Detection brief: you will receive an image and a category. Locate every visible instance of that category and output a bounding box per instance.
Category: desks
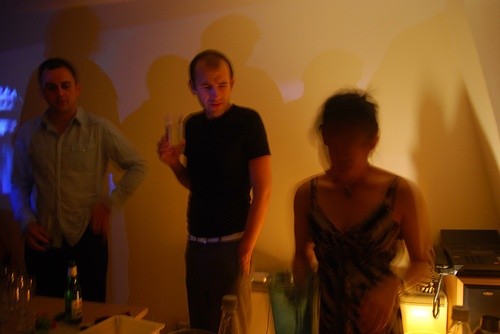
[0,297,149,334]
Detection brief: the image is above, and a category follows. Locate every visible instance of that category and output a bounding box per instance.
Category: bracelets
[397,278,405,295]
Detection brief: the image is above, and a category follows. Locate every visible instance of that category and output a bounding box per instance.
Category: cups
[165,113,183,153]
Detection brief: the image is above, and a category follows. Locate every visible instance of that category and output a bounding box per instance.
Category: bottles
[446,305,473,334]
[64,258,83,324]
[218,295,240,334]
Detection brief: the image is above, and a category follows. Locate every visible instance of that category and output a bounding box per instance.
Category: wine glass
[0,266,35,320]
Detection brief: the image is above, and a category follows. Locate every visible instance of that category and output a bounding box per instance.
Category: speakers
[456,283,500,334]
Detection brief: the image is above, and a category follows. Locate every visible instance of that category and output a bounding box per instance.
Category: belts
[189,231,245,243]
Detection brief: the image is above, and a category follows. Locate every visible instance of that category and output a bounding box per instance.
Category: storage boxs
[78,314,165,334]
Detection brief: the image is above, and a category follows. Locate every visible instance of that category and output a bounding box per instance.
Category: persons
[155,49,273,334]
[11,57,150,306]
[289,88,430,334]
